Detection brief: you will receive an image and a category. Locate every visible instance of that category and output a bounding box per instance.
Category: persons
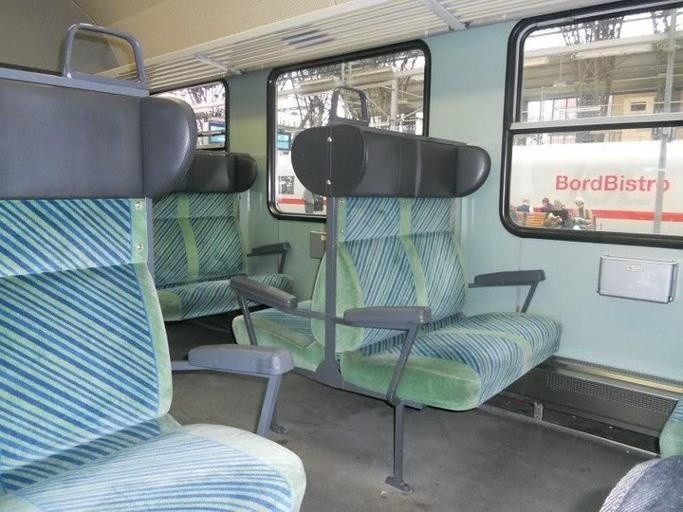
[572,199,593,230]
[301,188,314,214]
[539,197,555,212]
[540,199,571,229]
[514,197,534,212]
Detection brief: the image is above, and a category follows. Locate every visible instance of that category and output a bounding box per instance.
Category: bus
[196,112,309,219]
